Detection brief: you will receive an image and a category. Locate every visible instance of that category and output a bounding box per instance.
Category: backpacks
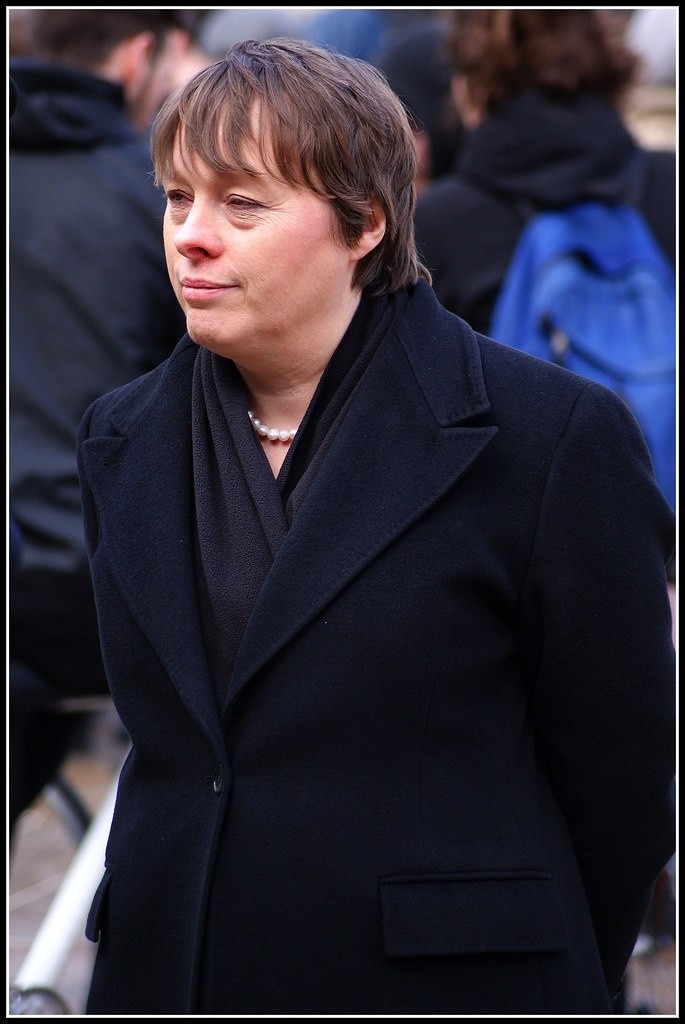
[486,147,676,514]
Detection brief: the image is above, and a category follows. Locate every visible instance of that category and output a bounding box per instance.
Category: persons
[379,28,478,198]
[8,6,192,868]
[413,10,681,965]
[74,36,677,1017]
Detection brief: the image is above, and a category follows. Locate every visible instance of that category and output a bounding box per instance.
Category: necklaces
[246,409,297,441]
